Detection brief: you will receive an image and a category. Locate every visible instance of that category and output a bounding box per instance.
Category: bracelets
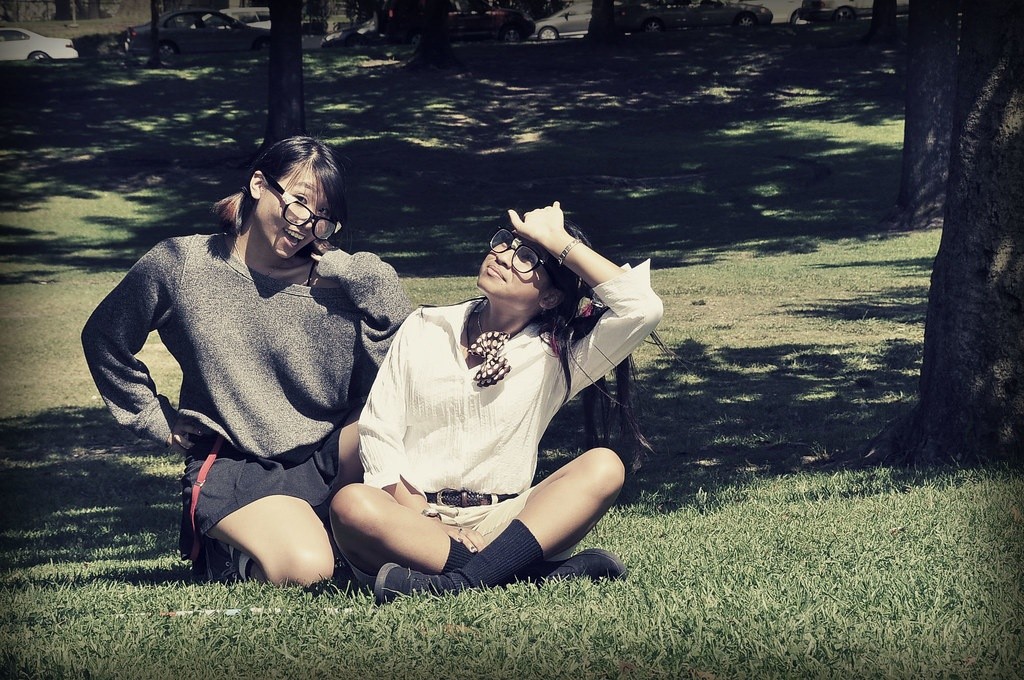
[558,239,583,266]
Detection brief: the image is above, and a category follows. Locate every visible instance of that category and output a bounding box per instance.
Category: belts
[424,491,518,507]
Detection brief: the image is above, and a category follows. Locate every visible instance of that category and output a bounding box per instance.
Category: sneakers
[374,563,443,604]
[205,537,244,585]
[535,549,627,590]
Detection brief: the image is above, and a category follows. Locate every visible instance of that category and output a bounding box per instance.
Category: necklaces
[477,313,484,334]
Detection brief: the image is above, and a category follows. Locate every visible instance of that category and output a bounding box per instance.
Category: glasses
[262,169,337,241]
[490,225,556,282]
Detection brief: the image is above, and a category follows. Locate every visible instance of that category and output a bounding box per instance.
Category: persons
[80,135,417,589]
[331,201,665,605]
